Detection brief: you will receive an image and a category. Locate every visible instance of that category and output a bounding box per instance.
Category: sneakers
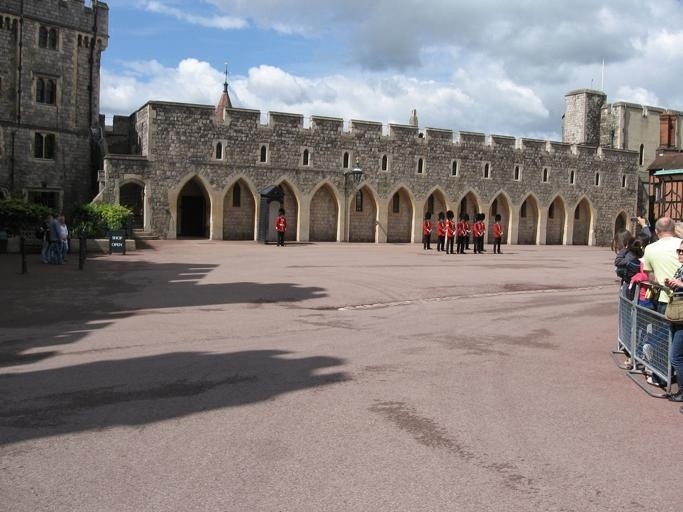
[615,356,683,403]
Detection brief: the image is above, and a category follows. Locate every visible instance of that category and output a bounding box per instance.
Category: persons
[608,212,682,415]
[37,213,69,265]
[272,213,288,247]
[421,218,504,254]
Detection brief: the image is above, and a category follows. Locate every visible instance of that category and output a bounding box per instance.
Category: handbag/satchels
[663,298,683,325]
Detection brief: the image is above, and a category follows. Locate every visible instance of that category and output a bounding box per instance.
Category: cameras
[630,217,639,225]
